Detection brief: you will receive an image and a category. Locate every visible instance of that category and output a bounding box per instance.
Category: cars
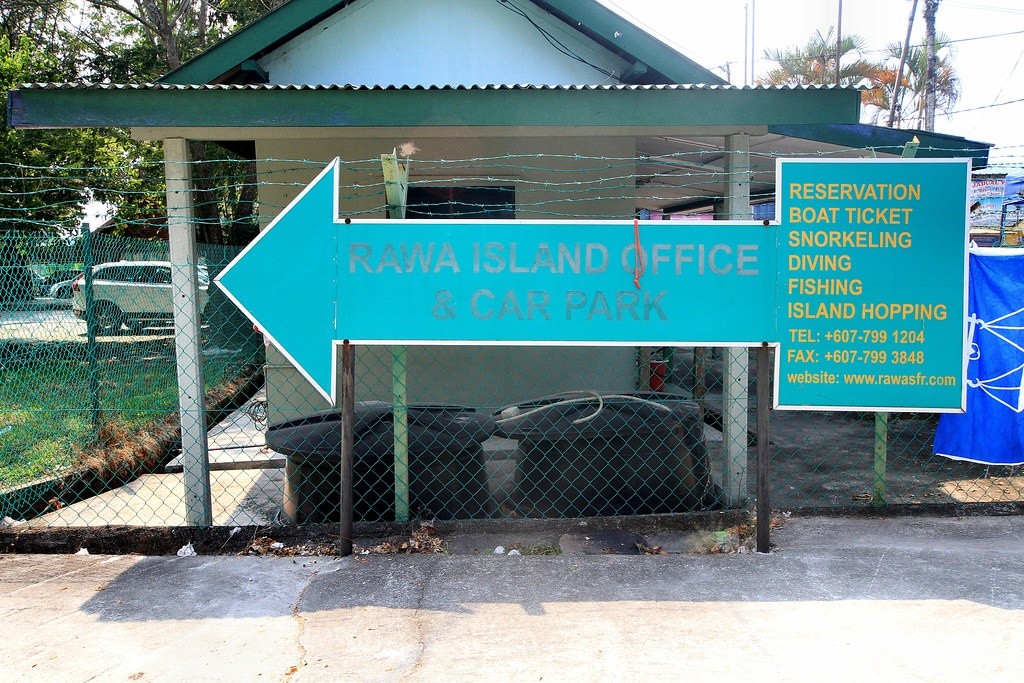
[25,269,86,299]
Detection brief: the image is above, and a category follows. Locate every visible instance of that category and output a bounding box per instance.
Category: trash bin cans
[262,402,497,526]
[490,394,723,515]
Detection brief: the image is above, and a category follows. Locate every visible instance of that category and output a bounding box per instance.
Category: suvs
[71,260,213,335]
